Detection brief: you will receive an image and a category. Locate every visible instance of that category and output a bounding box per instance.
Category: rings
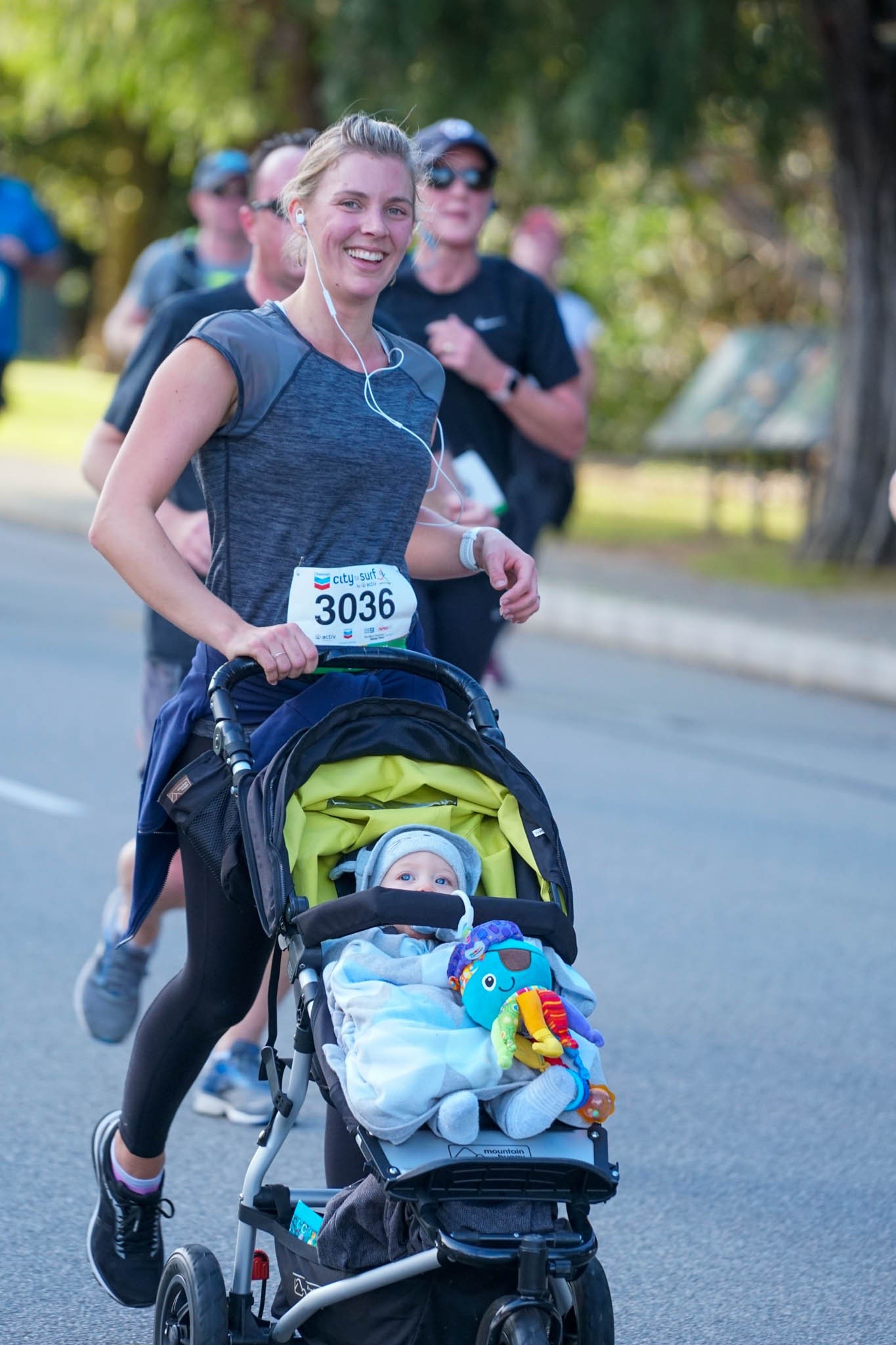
[274,651,287,659]
[537,593,541,601]
[442,341,454,354]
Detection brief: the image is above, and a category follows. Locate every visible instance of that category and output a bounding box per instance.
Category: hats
[412,117,499,173]
[188,148,249,190]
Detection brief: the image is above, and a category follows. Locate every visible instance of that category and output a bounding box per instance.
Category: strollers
[153,645,621,1344]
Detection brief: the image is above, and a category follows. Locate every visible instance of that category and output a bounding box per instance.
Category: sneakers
[192,1040,277,1124]
[77,889,155,1046]
[87,1107,174,1309]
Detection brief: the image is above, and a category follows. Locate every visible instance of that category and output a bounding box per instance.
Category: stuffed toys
[445,918,603,1079]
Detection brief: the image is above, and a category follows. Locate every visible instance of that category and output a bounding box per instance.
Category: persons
[89,114,543,1307]
[94,115,591,709]
[334,825,573,1143]
[46,114,507,1127]
[0,174,65,416]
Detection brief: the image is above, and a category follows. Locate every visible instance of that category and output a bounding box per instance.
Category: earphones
[296,214,305,224]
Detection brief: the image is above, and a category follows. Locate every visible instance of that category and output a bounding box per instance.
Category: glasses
[216,186,247,198]
[249,197,283,216]
[426,165,491,190]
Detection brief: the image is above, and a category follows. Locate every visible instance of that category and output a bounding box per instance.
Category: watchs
[488,367,520,403]
[458,526,487,572]
